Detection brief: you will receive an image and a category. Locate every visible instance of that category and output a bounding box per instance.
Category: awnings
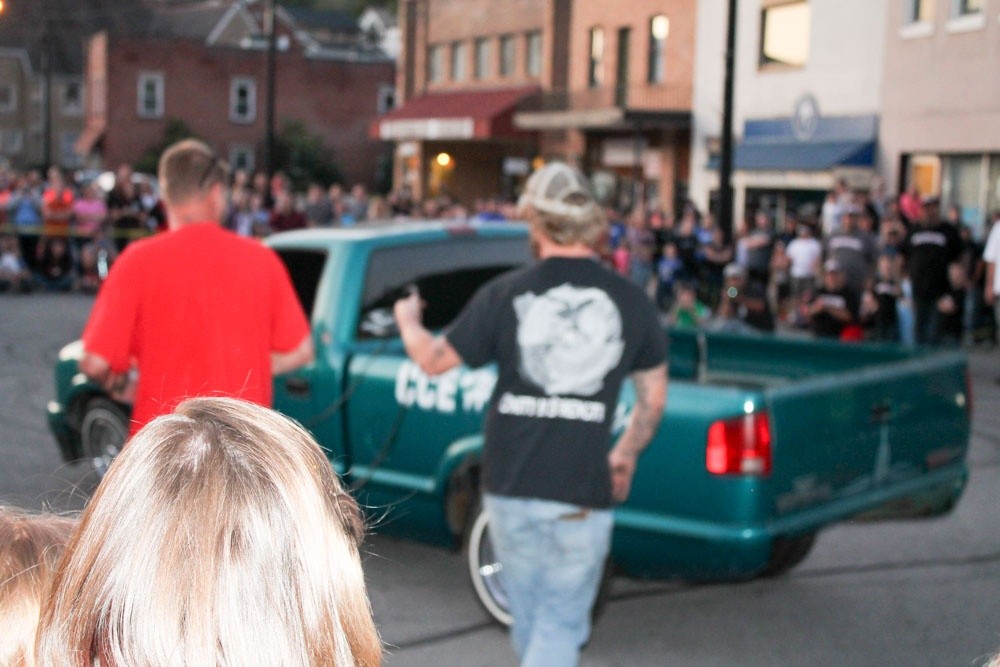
[704,115,877,171]
[371,86,543,141]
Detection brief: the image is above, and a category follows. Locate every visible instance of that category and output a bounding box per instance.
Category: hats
[515,161,595,217]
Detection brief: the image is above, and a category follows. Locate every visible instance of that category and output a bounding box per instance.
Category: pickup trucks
[41,218,977,637]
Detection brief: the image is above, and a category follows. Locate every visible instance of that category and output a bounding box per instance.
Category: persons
[393,160,668,667]
[81,138,314,441]
[606,178,999,389]
[0,396,383,667]
[0,157,516,296]
